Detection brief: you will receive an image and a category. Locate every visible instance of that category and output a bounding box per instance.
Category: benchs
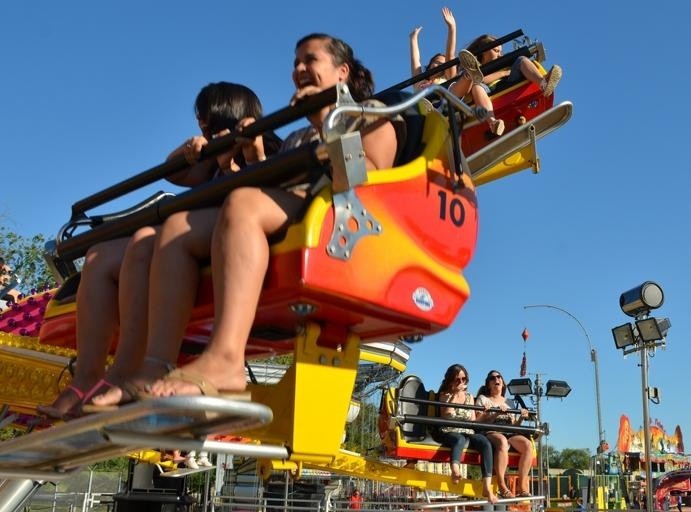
[39,91,479,368]
[379,375,541,469]
[376,29,572,190]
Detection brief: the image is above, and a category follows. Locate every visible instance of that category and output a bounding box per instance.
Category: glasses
[488,375,501,381]
[454,377,467,383]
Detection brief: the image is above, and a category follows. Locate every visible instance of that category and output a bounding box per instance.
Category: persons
[349,490,363,509]
[466,34,562,136]
[80,32,400,416]
[576,498,584,511]
[677,496,682,512]
[160,448,190,465]
[1,280,62,307]
[475,370,535,498]
[184,450,213,470]
[37,80,284,423]
[437,364,498,505]
[410,7,483,110]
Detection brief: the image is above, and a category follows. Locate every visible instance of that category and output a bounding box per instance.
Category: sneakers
[172,456,191,464]
[490,118,507,137]
[184,457,200,470]
[458,49,485,85]
[538,64,563,98]
[160,454,173,462]
[197,456,213,468]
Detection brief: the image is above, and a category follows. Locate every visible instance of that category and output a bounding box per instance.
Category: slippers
[516,490,531,498]
[39,379,87,419]
[137,367,254,408]
[68,377,119,417]
[499,488,515,499]
[79,380,145,414]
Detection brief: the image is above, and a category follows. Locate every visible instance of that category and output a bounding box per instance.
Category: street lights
[524,304,606,458]
[506,371,571,505]
[612,280,673,512]
